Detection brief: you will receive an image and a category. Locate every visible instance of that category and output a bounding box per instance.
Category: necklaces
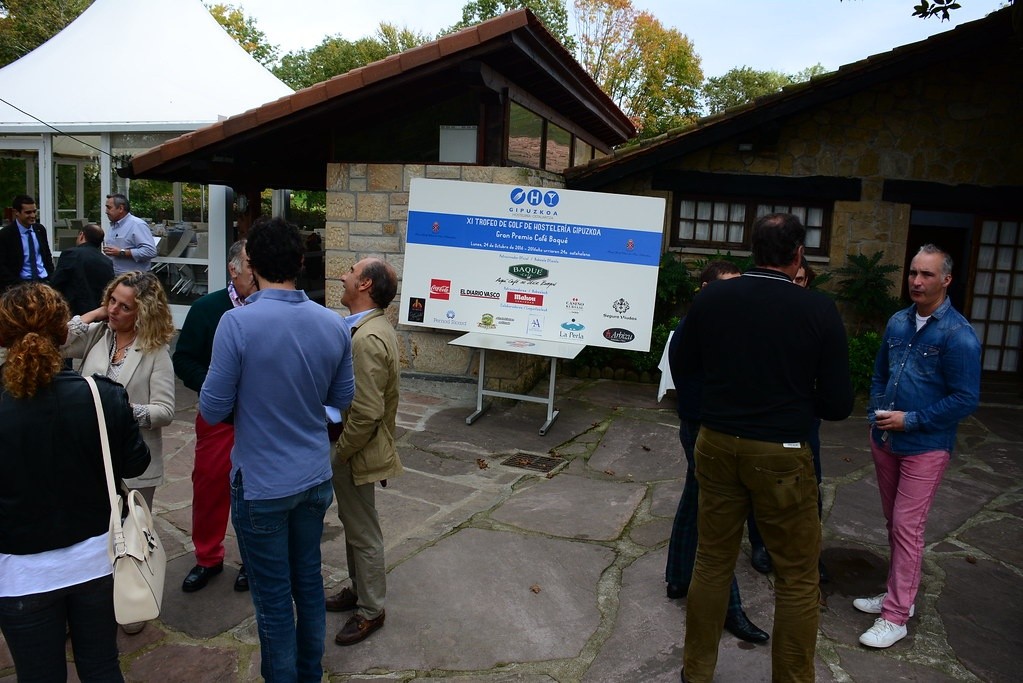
[114,336,136,360]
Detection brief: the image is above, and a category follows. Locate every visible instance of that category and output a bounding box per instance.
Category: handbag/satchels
[107,487,166,625]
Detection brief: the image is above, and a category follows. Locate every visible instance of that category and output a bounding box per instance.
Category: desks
[448,333,586,435]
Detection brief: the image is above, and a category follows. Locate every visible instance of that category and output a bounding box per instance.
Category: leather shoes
[182,560,224,592]
[722,610,770,643]
[234,565,250,591]
[666,577,691,599]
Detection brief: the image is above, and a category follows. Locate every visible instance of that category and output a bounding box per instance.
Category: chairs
[55,218,208,296]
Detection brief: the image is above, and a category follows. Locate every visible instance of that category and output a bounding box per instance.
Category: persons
[200,217,356,683]
[852,244,981,648]
[60,270,176,634]
[173,240,251,593]
[668,212,853,683]
[103,195,157,277]
[665,262,770,643]
[49,224,114,370]
[0,195,55,298]
[326,259,403,646]
[0,283,151,683]
[237,215,248,240]
[298,233,324,292]
[747,256,835,584]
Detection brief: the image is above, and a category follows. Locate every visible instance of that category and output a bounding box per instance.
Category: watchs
[121,248,125,257]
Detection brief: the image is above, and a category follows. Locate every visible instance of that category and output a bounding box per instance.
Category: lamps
[737,139,753,151]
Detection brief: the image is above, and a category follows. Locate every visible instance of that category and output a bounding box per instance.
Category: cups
[103,242,110,251]
[874,394,891,418]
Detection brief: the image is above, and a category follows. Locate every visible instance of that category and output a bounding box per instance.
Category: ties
[25,230,39,283]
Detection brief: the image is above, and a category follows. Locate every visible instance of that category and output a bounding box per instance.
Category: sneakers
[859,618,908,650]
[750,538,775,573]
[853,592,916,618]
[335,609,386,646]
[324,587,358,611]
[818,561,828,578]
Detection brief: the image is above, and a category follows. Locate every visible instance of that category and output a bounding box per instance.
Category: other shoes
[121,622,145,634]
